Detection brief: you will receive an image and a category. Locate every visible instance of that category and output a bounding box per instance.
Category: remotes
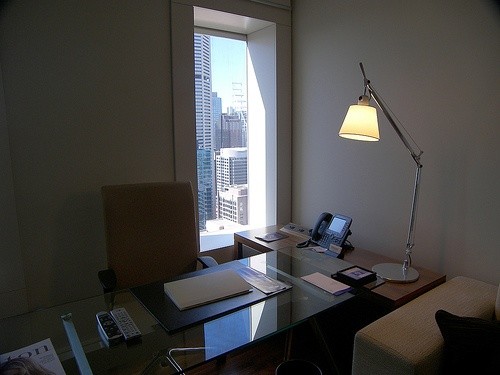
[96,311,122,340]
[111,308,142,339]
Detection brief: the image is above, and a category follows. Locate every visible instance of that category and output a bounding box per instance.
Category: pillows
[434,309,500,375]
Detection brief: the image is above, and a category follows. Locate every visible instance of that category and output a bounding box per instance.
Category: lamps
[338,61,424,283]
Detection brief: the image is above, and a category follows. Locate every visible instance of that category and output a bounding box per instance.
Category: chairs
[99,179,220,297]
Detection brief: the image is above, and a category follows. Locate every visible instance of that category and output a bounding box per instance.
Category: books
[0,338,66,375]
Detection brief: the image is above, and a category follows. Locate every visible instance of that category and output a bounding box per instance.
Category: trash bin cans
[276,359,323,375]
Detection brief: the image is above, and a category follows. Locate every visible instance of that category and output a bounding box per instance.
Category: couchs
[351,276,500,375]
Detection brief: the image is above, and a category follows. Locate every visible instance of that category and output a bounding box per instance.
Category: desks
[0,246,385,375]
[234,224,447,307]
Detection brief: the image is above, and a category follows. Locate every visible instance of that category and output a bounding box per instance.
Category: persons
[0,356,56,375]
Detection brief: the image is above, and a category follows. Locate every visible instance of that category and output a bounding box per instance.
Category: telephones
[310,212,352,249]
